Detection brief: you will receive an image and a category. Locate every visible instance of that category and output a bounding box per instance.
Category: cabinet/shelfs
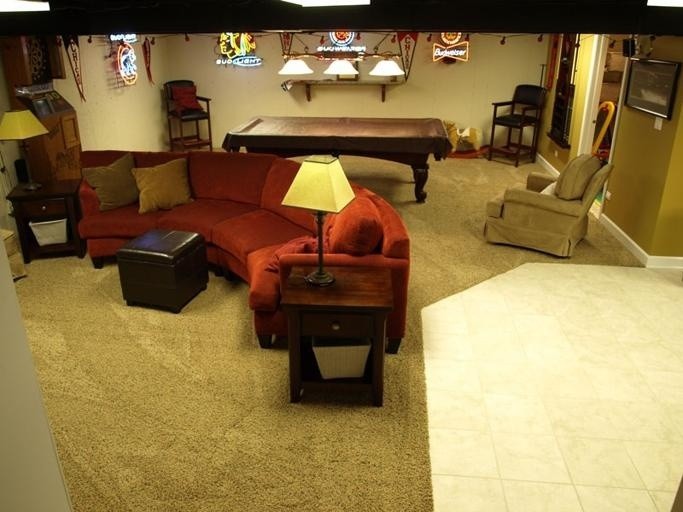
[6,177,87,264]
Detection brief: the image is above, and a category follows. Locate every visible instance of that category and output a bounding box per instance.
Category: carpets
[13,154,646,512]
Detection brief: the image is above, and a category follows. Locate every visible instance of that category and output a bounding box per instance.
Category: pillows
[131,157,194,215]
[324,190,383,254]
[266,234,317,272]
[81,152,140,211]
[540,180,557,196]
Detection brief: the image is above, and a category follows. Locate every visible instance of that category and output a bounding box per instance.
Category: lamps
[369,60,405,76]
[280,155,356,286]
[323,60,359,75]
[0,110,50,191]
[278,58,314,75]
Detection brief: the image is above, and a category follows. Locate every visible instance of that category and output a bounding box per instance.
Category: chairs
[163,79,213,152]
[489,84,547,167]
[482,153,614,257]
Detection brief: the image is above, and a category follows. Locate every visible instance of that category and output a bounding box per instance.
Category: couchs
[78,150,410,354]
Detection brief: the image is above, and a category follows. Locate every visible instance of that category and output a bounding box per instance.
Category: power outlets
[653,117,663,131]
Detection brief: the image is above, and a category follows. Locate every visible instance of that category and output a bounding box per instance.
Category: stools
[116,228,209,313]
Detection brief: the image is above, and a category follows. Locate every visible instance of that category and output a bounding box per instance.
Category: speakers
[623,39,635,57]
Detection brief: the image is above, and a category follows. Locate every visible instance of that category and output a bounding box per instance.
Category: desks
[222,115,454,203]
[280,265,394,407]
